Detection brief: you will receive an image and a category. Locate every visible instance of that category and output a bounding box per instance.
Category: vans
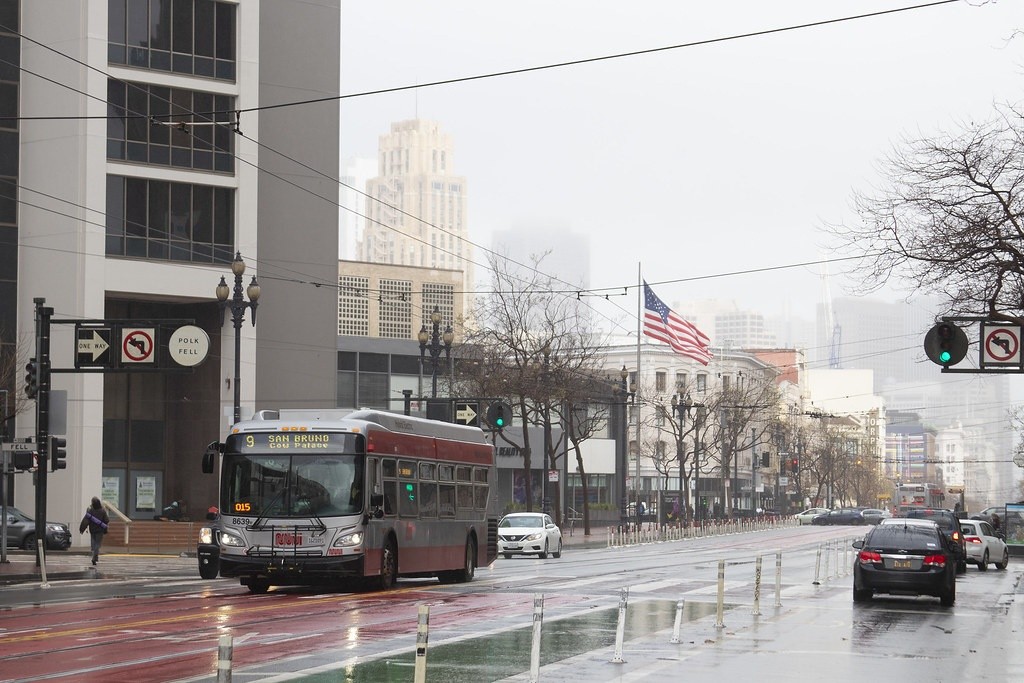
[906,506,970,574]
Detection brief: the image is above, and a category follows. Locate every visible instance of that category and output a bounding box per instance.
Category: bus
[202,407,499,593]
[896,484,945,520]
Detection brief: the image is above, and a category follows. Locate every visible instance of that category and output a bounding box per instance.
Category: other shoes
[91,559,98,565]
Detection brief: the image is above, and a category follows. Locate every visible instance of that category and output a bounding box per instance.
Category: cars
[793,507,893,526]
[497,513,563,559]
[852,523,958,606]
[957,520,1009,571]
[0,505,73,551]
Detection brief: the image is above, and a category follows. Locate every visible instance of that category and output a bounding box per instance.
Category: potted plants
[582,502,621,527]
[505,501,541,514]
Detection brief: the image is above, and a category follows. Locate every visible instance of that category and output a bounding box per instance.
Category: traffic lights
[792,458,798,473]
[486,402,513,428]
[924,321,968,367]
[25,358,36,399]
[51,437,66,471]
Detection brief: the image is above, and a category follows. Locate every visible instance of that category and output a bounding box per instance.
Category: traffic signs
[77,328,111,366]
[456,403,480,427]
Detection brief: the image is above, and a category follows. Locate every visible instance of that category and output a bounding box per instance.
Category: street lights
[418,303,454,397]
[671,382,692,521]
[727,411,745,515]
[613,365,639,533]
[216,249,261,426]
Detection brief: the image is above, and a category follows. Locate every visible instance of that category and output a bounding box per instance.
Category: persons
[334,473,362,513]
[759,505,766,516]
[79,496,109,565]
[989,514,1000,529]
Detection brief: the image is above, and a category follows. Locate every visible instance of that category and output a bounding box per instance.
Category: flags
[643,279,714,366]
